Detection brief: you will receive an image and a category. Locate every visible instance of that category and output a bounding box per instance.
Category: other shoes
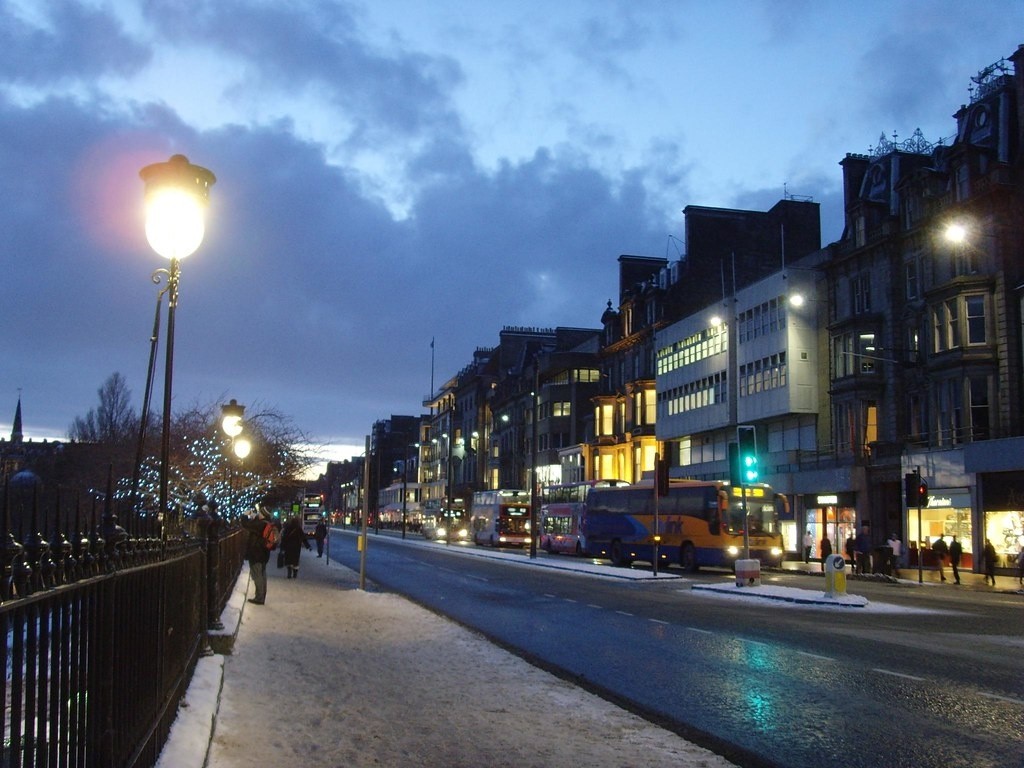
[248,599,264,605]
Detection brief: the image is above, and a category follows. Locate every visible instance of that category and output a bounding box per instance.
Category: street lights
[140,153,218,558]
[221,398,250,536]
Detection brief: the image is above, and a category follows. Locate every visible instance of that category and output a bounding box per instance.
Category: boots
[293,568,299,577]
[287,565,292,578]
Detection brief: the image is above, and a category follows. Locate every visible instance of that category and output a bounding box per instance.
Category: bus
[580,478,790,572]
[471,488,531,547]
[299,492,327,538]
[421,504,468,540]
[540,479,631,557]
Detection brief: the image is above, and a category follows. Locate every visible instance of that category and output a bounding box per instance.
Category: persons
[803,525,1024,586]
[271,516,312,578]
[314,519,327,558]
[241,505,272,605]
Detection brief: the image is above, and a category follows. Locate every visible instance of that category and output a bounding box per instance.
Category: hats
[259,506,272,516]
[208,500,219,510]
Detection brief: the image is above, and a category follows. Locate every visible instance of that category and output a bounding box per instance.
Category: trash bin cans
[855,552,870,573]
[873,546,894,574]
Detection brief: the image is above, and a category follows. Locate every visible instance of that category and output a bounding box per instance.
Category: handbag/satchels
[278,553,286,569]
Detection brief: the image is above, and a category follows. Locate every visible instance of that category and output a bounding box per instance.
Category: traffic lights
[736,425,758,485]
[919,485,928,506]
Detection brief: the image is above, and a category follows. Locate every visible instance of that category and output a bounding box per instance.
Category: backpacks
[262,520,279,550]
